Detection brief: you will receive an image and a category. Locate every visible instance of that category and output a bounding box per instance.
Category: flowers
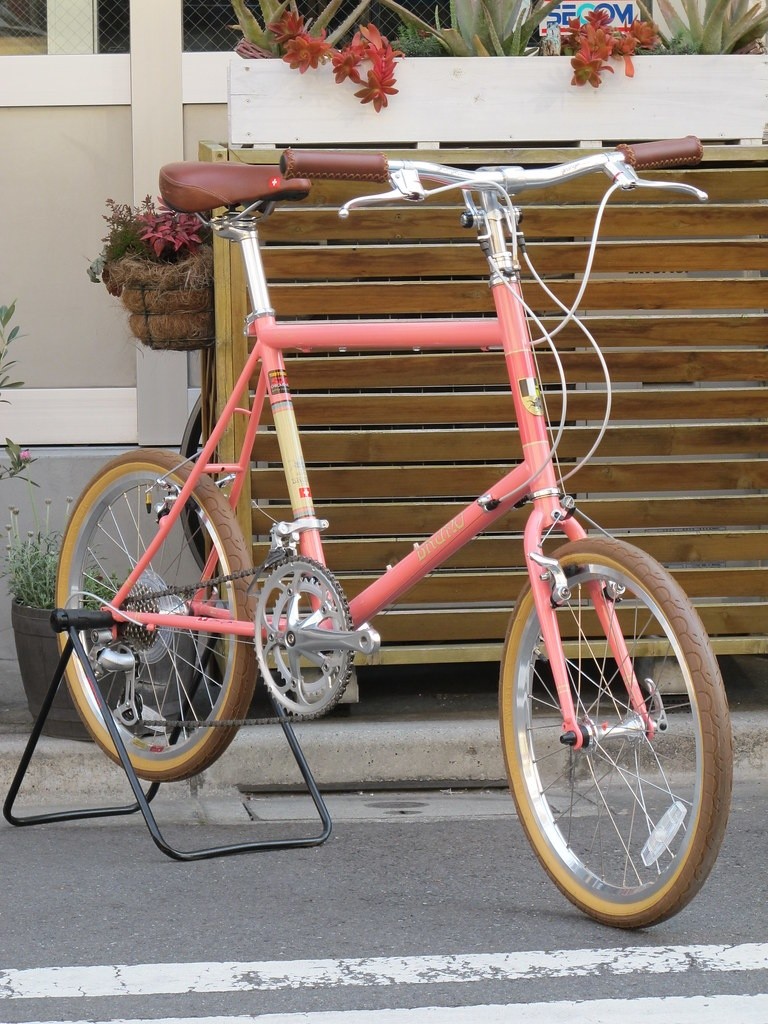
[2,450,140,612]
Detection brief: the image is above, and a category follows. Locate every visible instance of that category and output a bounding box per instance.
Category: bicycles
[3,133,733,930]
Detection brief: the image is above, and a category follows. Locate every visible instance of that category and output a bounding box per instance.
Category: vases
[10,591,126,744]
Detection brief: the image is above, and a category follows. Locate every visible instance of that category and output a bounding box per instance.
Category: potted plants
[226,0,768,145]
[100,192,218,353]
